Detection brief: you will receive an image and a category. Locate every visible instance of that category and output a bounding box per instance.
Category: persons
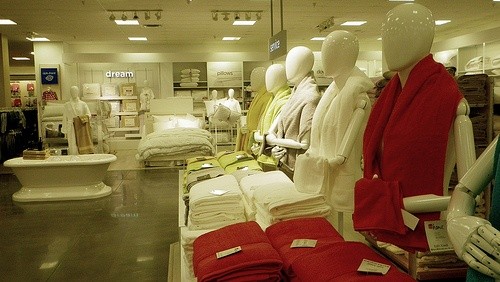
[266,46,321,172]
[42,87,59,105]
[363,3,476,234]
[211,90,218,101]
[224,88,241,114]
[251,63,291,156]
[297,30,371,204]
[140,80,154,103]
[240,66,271,148]
[445,133,500,282]
[61,86,91,155]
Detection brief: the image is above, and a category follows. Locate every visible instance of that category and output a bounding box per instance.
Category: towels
[23,149,50,160]
[247,83,292,156]
[258,70,321,178]
[73,115,94,154]
[293,65,376,212]
[182,151,417,282]
[234,87,272,154]
[352,54,464,253]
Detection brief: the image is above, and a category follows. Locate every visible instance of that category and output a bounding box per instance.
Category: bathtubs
[4,154,117,202]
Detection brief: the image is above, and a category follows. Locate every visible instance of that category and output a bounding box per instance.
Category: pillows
[186,113,196,118]
[181,74,200,77]
[180,69,200,74]
[177,118,200,129]
[181,77,199,82]
[153,119,175,131]
[211,103,242,126]
[152,114,175,122]
[170,115,202,125]
[179,82,198,87]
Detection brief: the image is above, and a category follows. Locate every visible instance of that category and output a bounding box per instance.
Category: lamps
[107,9,164,21]
[316,17,334,33]
[211,10,263,21]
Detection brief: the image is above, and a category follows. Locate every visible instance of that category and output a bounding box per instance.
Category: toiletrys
[27,137,47,151]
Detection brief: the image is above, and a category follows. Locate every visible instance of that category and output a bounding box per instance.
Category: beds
[135,113,217,167]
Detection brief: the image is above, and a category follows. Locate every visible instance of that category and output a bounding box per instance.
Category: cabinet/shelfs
[0,27,500,148]
[358,67,494,280]
